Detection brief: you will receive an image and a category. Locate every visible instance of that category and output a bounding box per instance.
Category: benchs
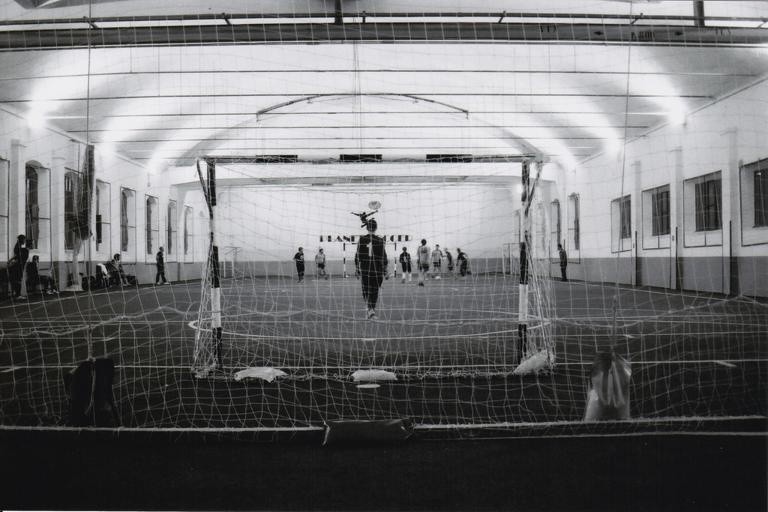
[96,263,136,288]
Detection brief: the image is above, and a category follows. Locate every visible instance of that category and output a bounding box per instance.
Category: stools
[26,279,36,292]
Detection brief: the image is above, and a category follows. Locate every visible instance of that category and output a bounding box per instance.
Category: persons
[110,254,132,287]
[28,255,61,295]
[352,209,470,319]
[315,248,326,280]
[14,235,27,300]
[154,246,171,285]
[294,247,304,283]
[558,243,569,282]
